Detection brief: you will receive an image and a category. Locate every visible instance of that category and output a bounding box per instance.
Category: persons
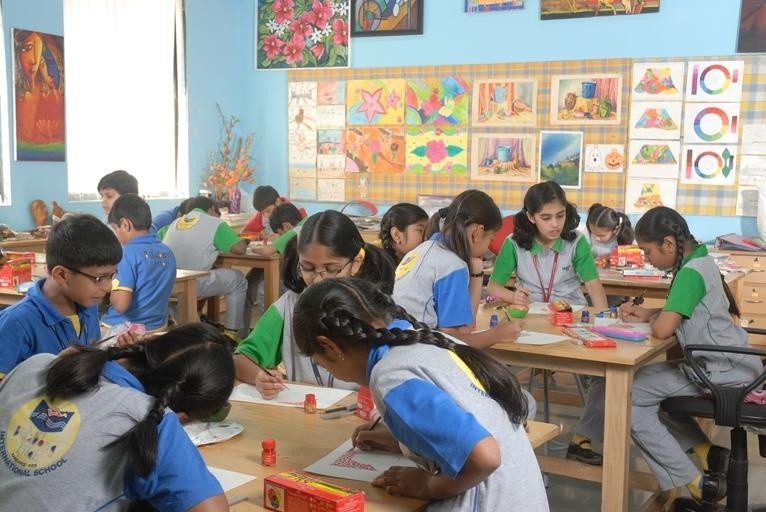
[1,214,138,376]
[368,203,430,265]
[98,169,139,234]
[391,190,536,420]
[487,180,608,465]
[581,203,634,258]
[1,322,235,512]
[292,276,551,511]
[618,206,763,512]
[100,193,177,330]
[233,209,395,400]
[152,185,309,344]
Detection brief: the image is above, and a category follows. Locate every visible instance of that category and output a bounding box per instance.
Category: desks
[185,377,562,512]
[0,251,211,325]
[470,298,680,512]
[0,239,47,253]
[482,257,753,325]
[207,245,283,322]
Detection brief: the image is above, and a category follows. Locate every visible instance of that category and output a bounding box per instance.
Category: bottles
[228,183,241,214]
[490,315,498,328]
[303,394,316,415]
[609,307,618,319]
[580,310,589,323]
[261,439,277,466]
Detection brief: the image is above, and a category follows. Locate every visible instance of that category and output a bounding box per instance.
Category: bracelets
[470,271,484,277]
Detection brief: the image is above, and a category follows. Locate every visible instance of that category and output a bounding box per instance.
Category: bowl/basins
[199,402,231,423]
[505,304,529,320]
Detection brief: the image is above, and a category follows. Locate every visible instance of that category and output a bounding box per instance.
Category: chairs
[661,328,766,512]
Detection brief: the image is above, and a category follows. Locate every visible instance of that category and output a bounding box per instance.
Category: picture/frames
[351,0,424,37]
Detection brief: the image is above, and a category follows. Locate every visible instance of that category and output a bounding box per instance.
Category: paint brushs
[354,416,381,448]
[96,335,117,345]
[239,351,290,391]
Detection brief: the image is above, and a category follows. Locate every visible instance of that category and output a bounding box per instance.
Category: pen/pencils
[616,289,649,323]
[502,307,511,321]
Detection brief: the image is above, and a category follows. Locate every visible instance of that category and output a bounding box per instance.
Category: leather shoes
[703,446,731,477]
[692,476,727,507]
[567,440,602,465]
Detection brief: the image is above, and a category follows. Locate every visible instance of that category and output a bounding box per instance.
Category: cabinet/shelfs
[707,244,766,346]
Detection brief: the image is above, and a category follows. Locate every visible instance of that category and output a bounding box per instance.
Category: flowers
[203,102,257,194]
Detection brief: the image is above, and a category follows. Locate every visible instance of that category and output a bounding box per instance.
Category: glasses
[64,265,118,285]
[296,259,352,280]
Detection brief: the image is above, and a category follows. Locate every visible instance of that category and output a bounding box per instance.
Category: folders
[714,233,762,252]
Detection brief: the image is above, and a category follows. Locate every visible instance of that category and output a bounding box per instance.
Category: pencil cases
[597,326,646,342]
